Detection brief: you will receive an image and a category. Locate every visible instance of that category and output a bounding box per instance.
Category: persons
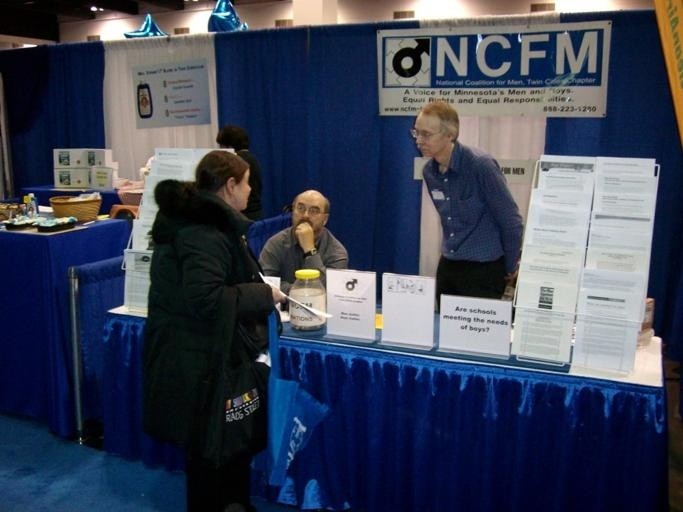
[408,101,524,312]
[212,121,265,217]
[139,147,286,511]
[254,187,348,301]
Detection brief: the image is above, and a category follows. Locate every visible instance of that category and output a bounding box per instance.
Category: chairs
[244,214,293,260]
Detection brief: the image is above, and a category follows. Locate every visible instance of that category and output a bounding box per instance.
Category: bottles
[25,193,39,217]
[137,78,153,119]
[288,268,327,332]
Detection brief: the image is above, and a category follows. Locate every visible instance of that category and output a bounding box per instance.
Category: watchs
[301,249,318,258]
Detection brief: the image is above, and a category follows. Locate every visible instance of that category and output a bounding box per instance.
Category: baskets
[48,193,103,224]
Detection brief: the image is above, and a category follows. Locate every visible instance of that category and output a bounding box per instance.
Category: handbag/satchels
[201,359,277,475]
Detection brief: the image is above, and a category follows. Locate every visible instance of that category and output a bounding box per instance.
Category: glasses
[296,205,326,216]
[409,127,446,141]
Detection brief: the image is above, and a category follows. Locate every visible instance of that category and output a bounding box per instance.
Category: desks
[99,301,669,512]
[21,185,121,215]
[0,211,132,437]
